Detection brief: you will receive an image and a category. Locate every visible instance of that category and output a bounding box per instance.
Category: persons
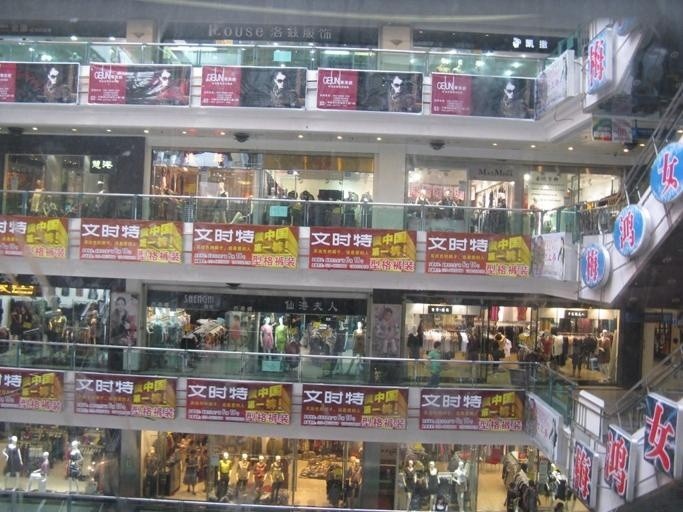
[435,58,464,74]
[507,471,563,512]
[348,456,363,508]
[67,440,83,495]
[163,40,178,64]
[387,74,415,112]
[43,67,71,102]
[671,338,682,378]
[403,460,468,512]
[110,46,121,63]
[500,80,529,118]
[25,452,49,492]
[2,436,23,492]
[146,69,188,105]
[180,433,211,495]
[217,453,285,504]
[270,70,300,108]
